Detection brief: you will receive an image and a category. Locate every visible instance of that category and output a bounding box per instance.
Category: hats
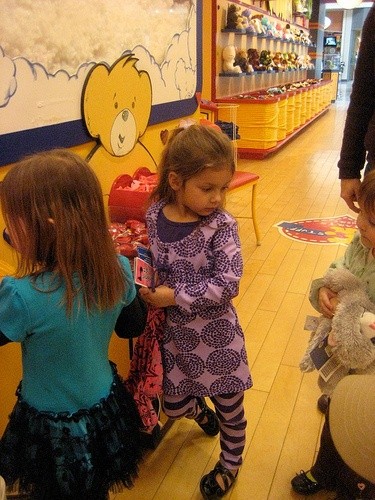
[329,374,375,483]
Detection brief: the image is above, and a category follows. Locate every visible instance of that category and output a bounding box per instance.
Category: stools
[199,119,261,246]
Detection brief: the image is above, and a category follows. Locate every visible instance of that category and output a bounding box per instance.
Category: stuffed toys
[222,3,319,100]
[299,266,375,397]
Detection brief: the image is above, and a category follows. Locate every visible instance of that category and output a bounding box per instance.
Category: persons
[0,149,147,500]
[291,168,375,500]
[337,2,375,212]
[137,124,254,500]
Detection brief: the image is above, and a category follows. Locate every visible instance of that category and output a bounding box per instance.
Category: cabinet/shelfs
[219,4,316,77]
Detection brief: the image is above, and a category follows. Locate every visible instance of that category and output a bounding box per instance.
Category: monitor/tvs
[324,37,337,46]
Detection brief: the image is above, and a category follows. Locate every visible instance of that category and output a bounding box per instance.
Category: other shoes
[193,398,221,436]
[199,460,239,500]
[291,469,326,494]
